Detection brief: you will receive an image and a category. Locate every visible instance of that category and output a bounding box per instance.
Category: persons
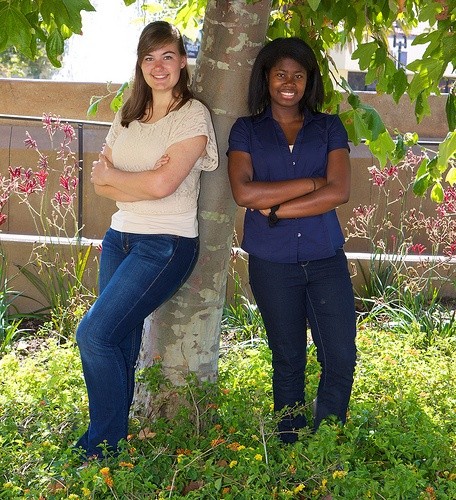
[226,37,357,444]
[73,22,218,466]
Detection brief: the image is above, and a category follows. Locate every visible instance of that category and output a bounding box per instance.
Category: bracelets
[269,204,280,224]
[308,178,316,191]
